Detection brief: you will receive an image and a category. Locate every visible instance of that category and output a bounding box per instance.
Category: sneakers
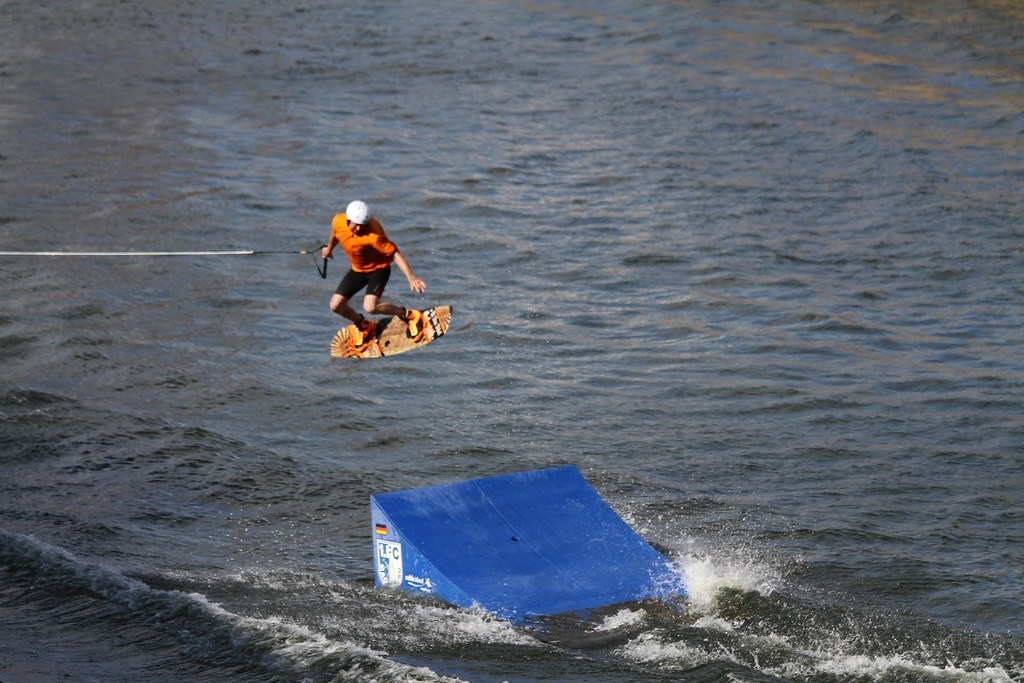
[354,319,374,348]
[400,307,426,342]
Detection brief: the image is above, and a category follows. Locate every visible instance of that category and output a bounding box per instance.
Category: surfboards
[329,303,453,360]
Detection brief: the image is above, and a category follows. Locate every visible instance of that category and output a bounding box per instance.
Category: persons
[322,200,426,352]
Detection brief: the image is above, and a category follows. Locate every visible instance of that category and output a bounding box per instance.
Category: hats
[346,200,369,224]
